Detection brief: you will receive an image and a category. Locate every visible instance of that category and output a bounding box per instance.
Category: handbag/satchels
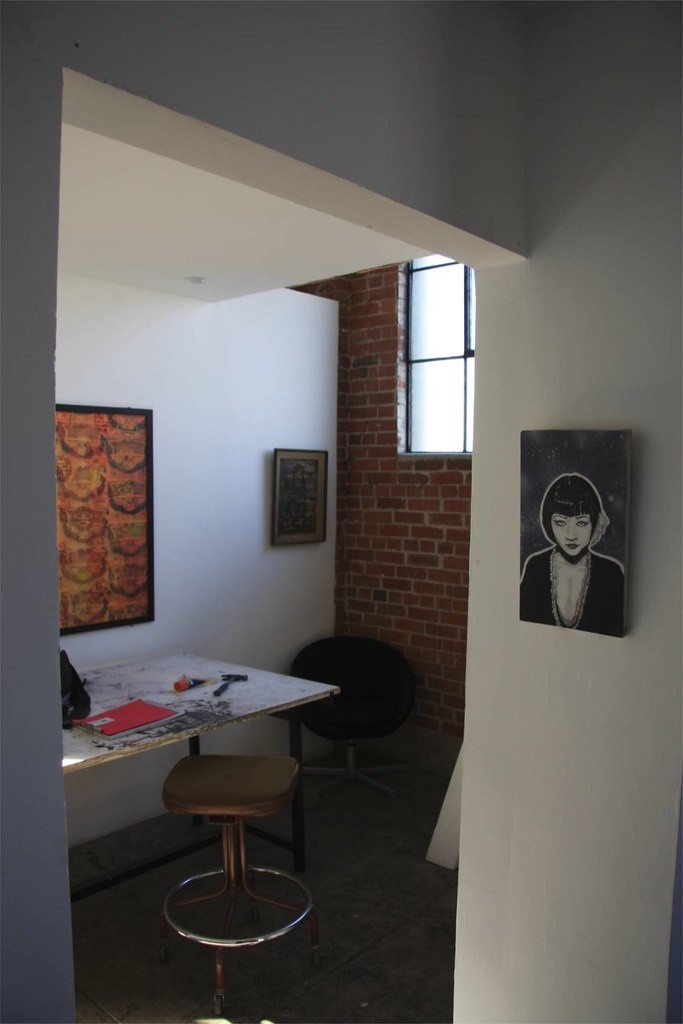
[60,649,91,729]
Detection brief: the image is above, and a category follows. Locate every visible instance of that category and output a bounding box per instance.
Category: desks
[58,652,340,901]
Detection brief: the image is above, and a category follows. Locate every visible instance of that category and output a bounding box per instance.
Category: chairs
[289,636,432,798]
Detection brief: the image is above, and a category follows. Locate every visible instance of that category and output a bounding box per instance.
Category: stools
[162,755,321,1013]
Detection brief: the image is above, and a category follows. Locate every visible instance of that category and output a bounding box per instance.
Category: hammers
[213,674,247,696]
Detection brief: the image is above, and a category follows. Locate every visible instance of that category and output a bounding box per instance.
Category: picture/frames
[56,402,156,640]
[269,448,329,545]
